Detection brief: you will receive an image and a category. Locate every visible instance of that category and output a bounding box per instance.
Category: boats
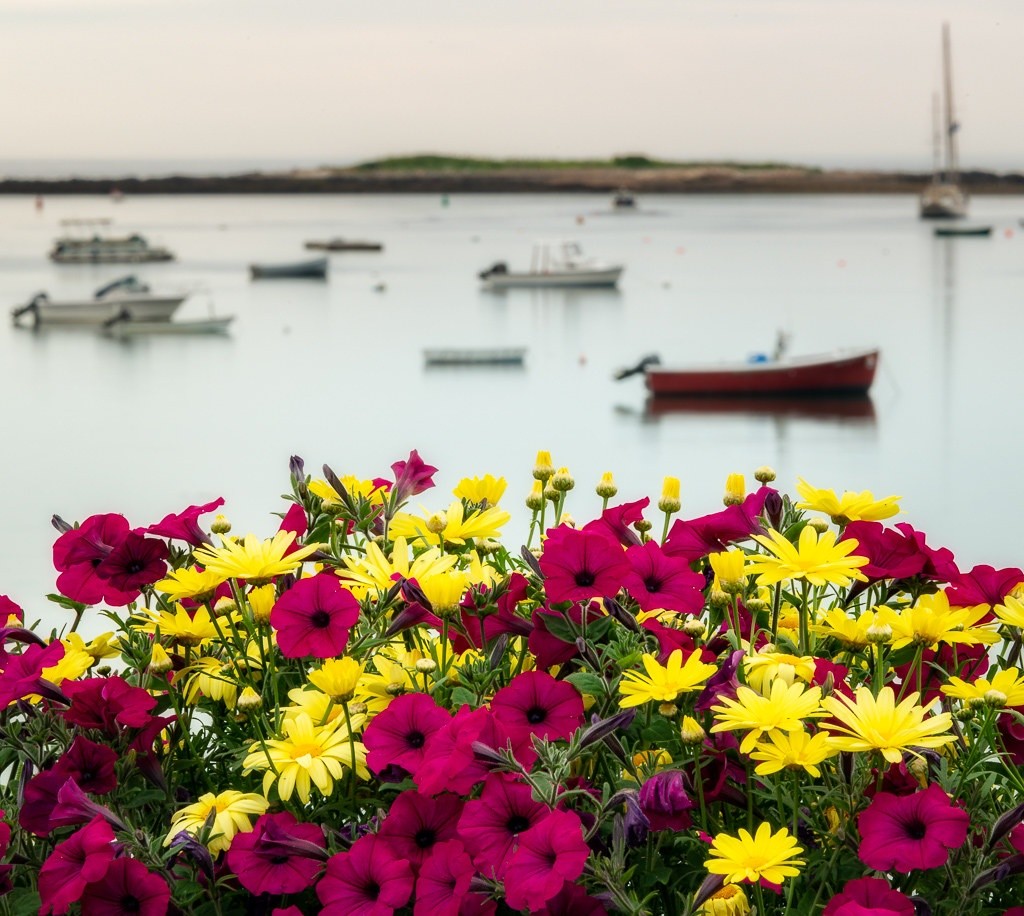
[646,347,877,398]
[612,190,635,205]
[934,224,990,236]
[48,235,171,265]
[422,343,525,366]
[108,314,229,335]
[32,291,193,322]
[481,263,622,286]
[303,236,381,251]
[248,254,329,279]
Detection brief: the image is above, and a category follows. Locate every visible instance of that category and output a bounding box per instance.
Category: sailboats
[920,22,967,215]
